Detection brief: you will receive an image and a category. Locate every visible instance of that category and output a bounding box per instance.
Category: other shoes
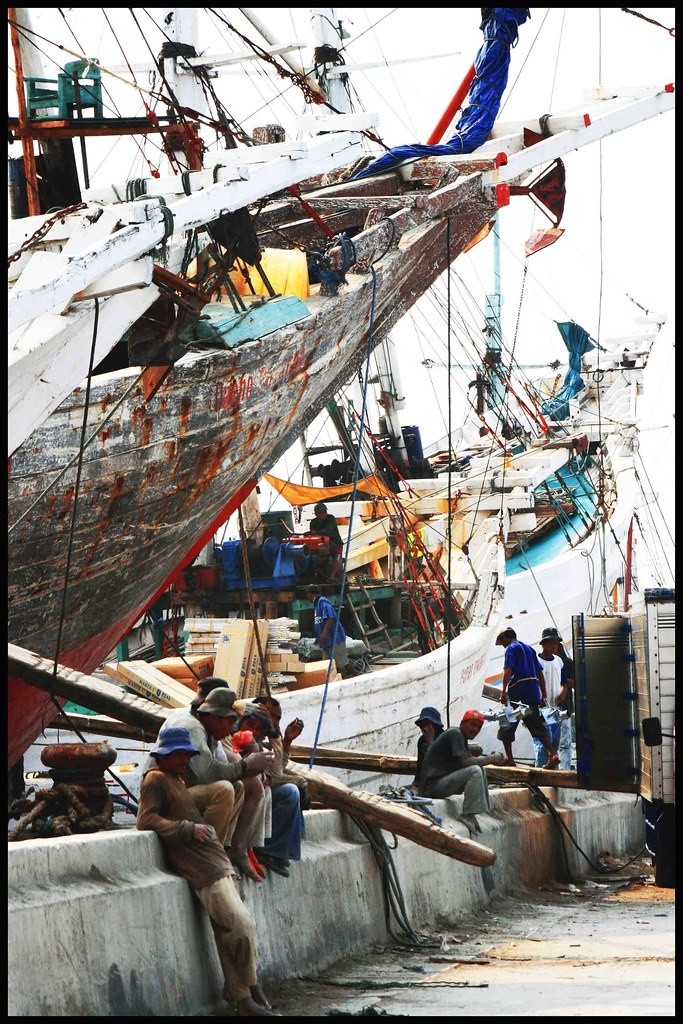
[254,850,291,878]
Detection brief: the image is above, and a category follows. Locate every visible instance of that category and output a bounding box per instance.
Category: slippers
[543,756,561,769]
[497,759,516,766]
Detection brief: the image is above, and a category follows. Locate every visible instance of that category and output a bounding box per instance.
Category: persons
[555,645,575,769]
[419,710,489,838]
[309,502,347,579]
[535,629,564,767]
[308,584,347,669]
[496,627,561,768]
[145,677,304,882]
[138,726,280,1017]
[413,707,447,793]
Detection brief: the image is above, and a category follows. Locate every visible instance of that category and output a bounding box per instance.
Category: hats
[197,688,246,724]
[539,627,563,645]
[463,710,485,725]
[414,707,444,727]
[495,626,517,645]
[315,503,329,512]
[242,702,272,731]
[149,726,201,758]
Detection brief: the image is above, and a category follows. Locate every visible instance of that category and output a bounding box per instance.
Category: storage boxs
[104,619,343,710]
[175,565,217,592]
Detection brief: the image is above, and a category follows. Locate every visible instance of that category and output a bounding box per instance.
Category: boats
[9,5,675,724]
[215,325,666,706]
[17,501,506,830]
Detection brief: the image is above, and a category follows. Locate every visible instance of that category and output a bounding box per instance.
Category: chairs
[23,58,107,121]
